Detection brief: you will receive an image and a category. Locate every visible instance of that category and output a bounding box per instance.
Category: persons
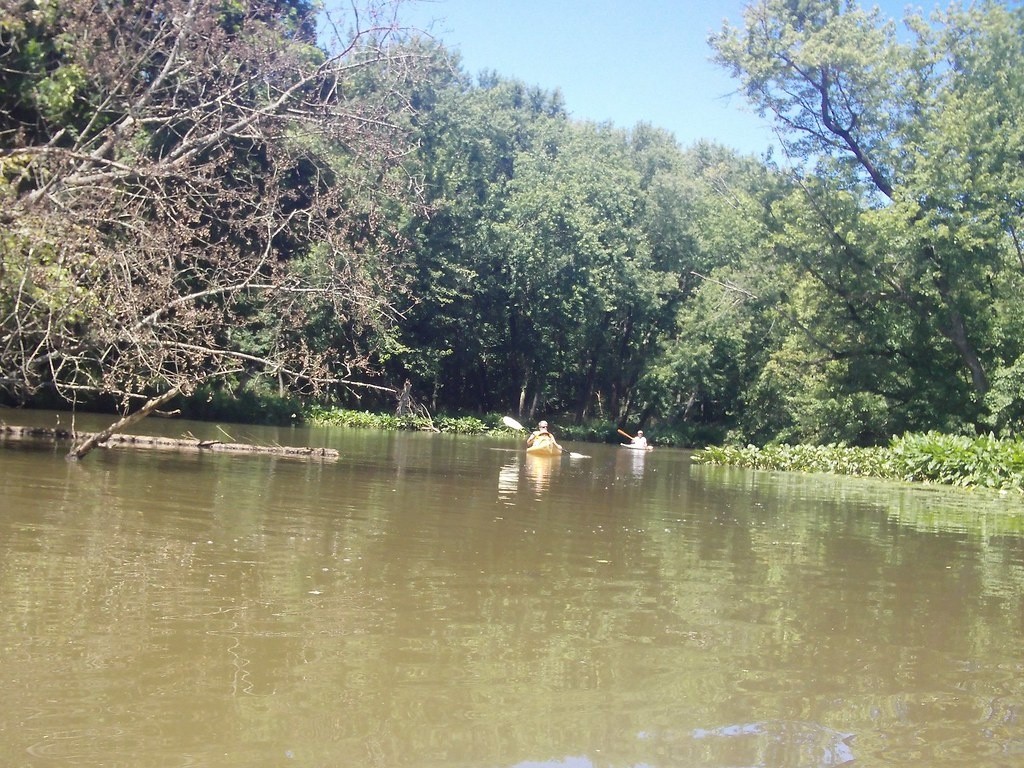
[632,430,647,446]
[527,421,562,449]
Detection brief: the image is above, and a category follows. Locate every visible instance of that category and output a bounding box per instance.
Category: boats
[620,443,654,450]
[526,433,562,455]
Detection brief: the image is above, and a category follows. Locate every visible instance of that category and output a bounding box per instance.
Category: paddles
[503,416,582,458]
[617,429,634,441]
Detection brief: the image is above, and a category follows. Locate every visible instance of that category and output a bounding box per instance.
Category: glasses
[539,426,548,428]
